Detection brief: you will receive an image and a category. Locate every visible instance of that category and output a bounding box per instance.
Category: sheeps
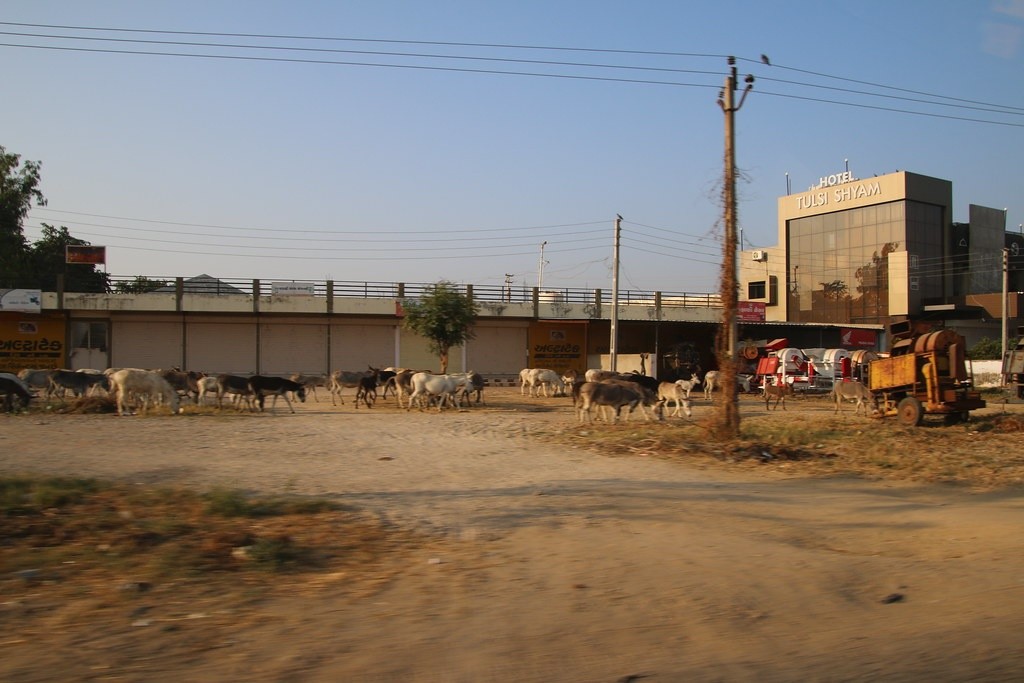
[0,364,754,426]
[762,381,796,412]
[831,379,877,418]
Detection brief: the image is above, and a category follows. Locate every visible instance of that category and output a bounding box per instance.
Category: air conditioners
[751,250,764,261]
